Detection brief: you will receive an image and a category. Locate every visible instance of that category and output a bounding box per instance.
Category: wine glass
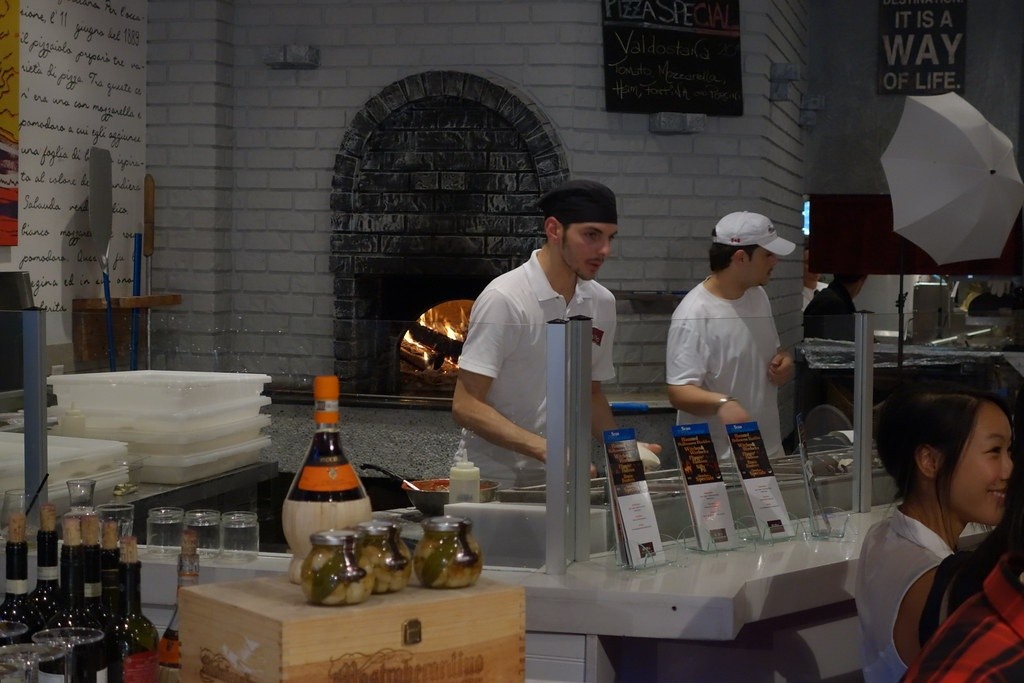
[0,621,104,683]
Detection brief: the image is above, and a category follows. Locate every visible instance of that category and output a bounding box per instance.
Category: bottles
[358,520,412,593]
[0,513,46,683]
[160,530,199,683]
[147,507,183,557]
[222,511,259,563]
[29,505,61,625]
[301,531,375,605]
[38,513,160,683]
[282,376,372,584]
[185,510,221,560]
[414,515,484,589]
[449,449,480,504]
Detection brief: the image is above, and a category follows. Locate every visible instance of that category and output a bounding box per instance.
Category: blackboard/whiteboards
[601,0,744,116]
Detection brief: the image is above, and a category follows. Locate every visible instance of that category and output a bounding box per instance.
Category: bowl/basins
[402,479,502,517]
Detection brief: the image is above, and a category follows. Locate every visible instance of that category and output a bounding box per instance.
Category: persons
[451,180,662,489]
[854,377,1024,683]
[666,210,796,462]
[803,235,829,311]
[803,273,879,344]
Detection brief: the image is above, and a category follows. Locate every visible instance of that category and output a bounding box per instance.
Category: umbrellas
[879,91,1024,265]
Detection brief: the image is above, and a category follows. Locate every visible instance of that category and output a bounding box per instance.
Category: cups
[67,479,96,510]
[0,489,47,552]
[97,505,134,547]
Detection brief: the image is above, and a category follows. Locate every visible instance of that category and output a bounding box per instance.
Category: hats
[539,179,617,224]
[712,210,797,256]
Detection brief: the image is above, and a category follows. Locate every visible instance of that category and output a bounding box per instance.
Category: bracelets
[714,396,737,411]
